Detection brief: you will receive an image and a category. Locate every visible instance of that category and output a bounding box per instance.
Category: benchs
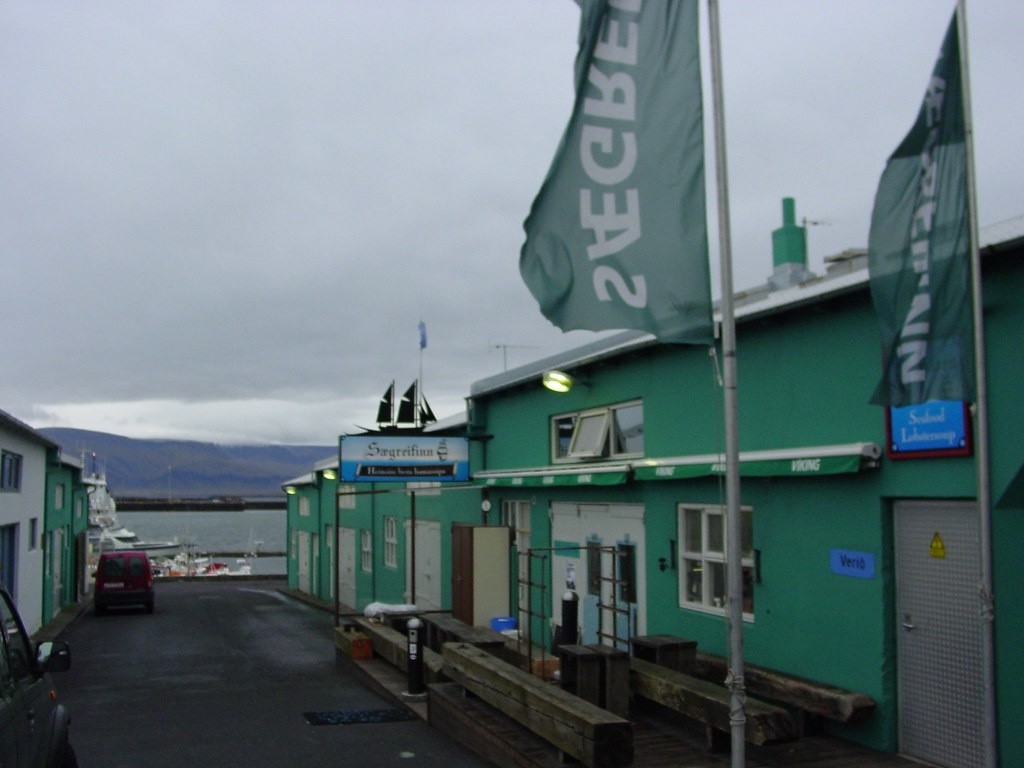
[629,657,800,754]
[476,625,560,680]
[442,642,634,768]
[356,617,452,687]
[695,652,876,738]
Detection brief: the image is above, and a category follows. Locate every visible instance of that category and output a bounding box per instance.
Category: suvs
[0,584,79,768]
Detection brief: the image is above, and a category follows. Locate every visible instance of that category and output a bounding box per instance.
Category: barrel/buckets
[490,618,518,633]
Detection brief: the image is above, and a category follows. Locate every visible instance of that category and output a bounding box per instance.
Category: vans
[91,550,161,612]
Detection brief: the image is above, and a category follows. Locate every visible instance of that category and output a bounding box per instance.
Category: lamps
[285,478,296,494]
[542,370,593,393]
[323,470,337,480]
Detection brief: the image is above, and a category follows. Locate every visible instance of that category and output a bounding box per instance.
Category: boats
[73,442,265,577]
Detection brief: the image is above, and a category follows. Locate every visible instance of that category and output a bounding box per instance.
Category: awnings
[633,442,881,482]
[472,462,631,489]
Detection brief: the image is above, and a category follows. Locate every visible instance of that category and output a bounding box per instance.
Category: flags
[865,6,969,406]
[519,0,716,346]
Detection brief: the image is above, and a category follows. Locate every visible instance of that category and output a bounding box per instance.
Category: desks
[421,612,505,658]
[630,633,699,676]
[560,643,630,718]
